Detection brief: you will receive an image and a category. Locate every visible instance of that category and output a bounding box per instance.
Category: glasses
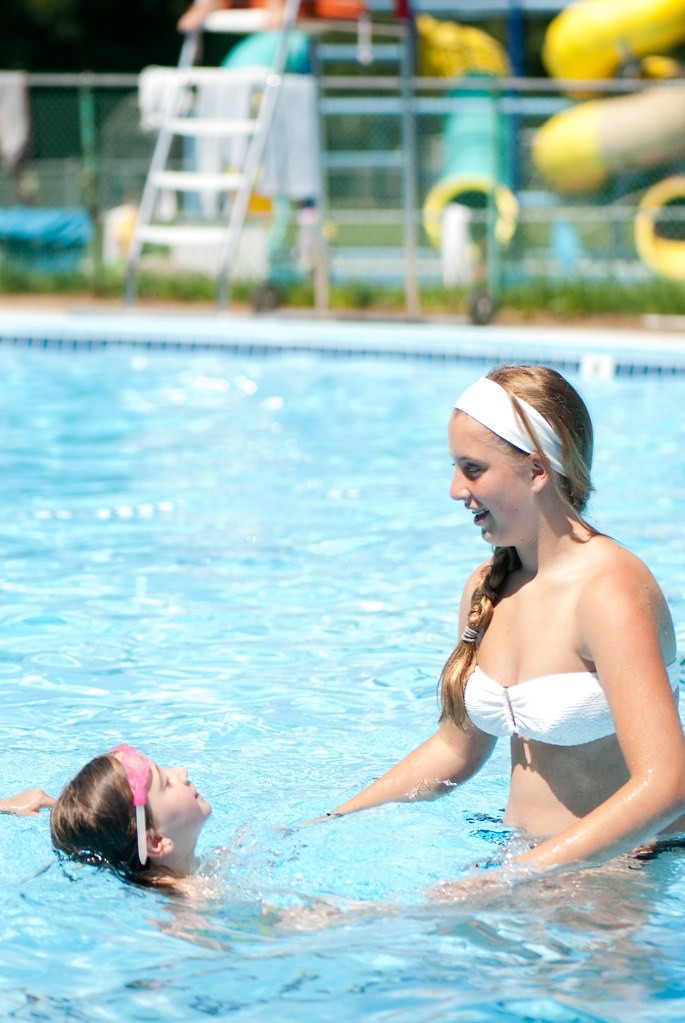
[110,743,149,867]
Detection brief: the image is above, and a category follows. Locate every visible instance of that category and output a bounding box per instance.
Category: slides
[532,0,685,284]
[415,10,519,260]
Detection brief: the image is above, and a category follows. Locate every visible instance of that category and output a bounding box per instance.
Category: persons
[54,745,213,876]
[336,364,685,869]
